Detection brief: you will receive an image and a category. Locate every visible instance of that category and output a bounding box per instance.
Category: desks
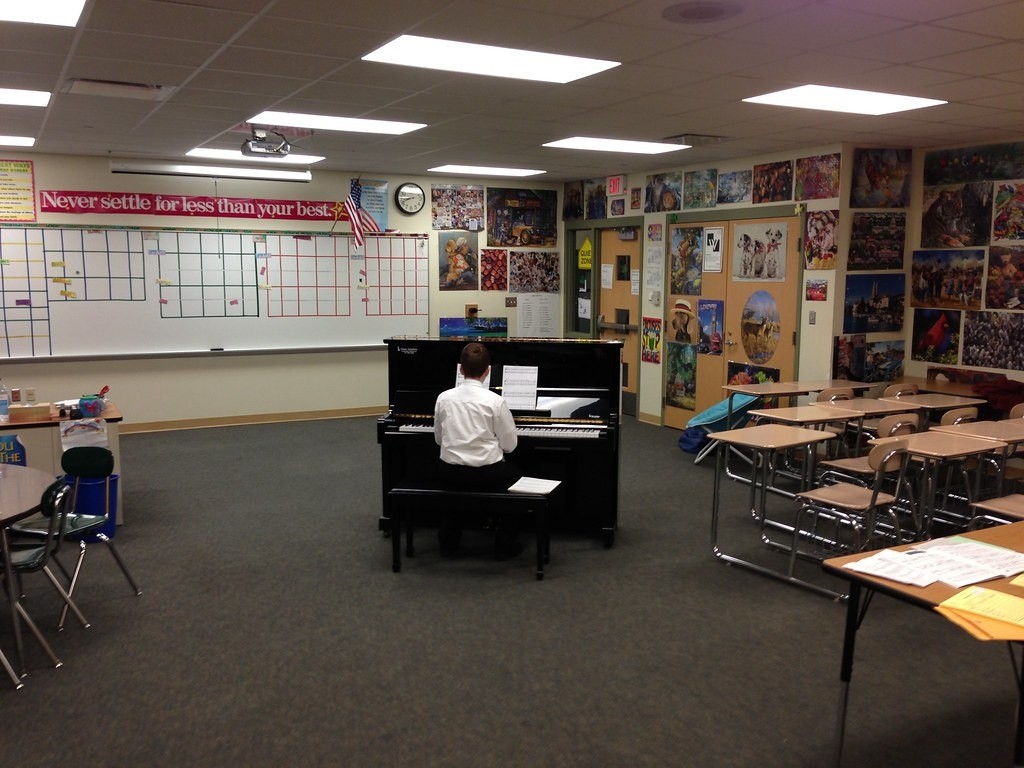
[782,378,879,407]
[0,464,58,679]
[866,431,1008,544]
[878,393,989,432]
[0,400,124,531]
[720,383,829,500]
[705,423,850,604]
[928,420,1024,523]
[822,519,1024,768]
[807,399,921,484]
[891,376,985,422]
[747,405,866,499]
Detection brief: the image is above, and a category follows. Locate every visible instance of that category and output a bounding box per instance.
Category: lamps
[108,158,312,184]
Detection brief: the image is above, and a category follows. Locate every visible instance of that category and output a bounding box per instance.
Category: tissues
[8,402,50,422]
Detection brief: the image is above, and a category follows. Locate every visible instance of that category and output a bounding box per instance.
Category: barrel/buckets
[56,474,120,543]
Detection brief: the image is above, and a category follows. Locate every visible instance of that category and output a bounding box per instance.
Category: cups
[93,394,106,411]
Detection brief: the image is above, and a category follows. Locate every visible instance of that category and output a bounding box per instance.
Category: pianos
[374,333,624,547]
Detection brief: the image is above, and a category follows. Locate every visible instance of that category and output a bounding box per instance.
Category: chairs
[0,474,92,670]
[7,447,143,633]
[787,383,1024,577]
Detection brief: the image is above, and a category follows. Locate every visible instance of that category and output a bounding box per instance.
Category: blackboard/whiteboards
[0,222,431,364]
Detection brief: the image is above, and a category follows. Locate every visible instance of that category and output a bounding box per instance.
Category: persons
[670,299,695,343]
[434,343,525,563]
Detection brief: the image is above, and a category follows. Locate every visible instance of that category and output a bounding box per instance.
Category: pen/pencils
[210,348,224,351]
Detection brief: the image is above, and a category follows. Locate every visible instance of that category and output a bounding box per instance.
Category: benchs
[387,475,568,583]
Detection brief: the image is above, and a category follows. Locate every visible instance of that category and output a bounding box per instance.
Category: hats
[456,237,467,250]
[670,299,696,320]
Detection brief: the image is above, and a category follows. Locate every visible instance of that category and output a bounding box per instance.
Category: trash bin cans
[56,473,120,543]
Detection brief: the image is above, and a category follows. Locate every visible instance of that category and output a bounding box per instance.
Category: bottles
[0,378,9,423]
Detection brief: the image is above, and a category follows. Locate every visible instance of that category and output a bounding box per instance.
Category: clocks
[394,182,426,215]
